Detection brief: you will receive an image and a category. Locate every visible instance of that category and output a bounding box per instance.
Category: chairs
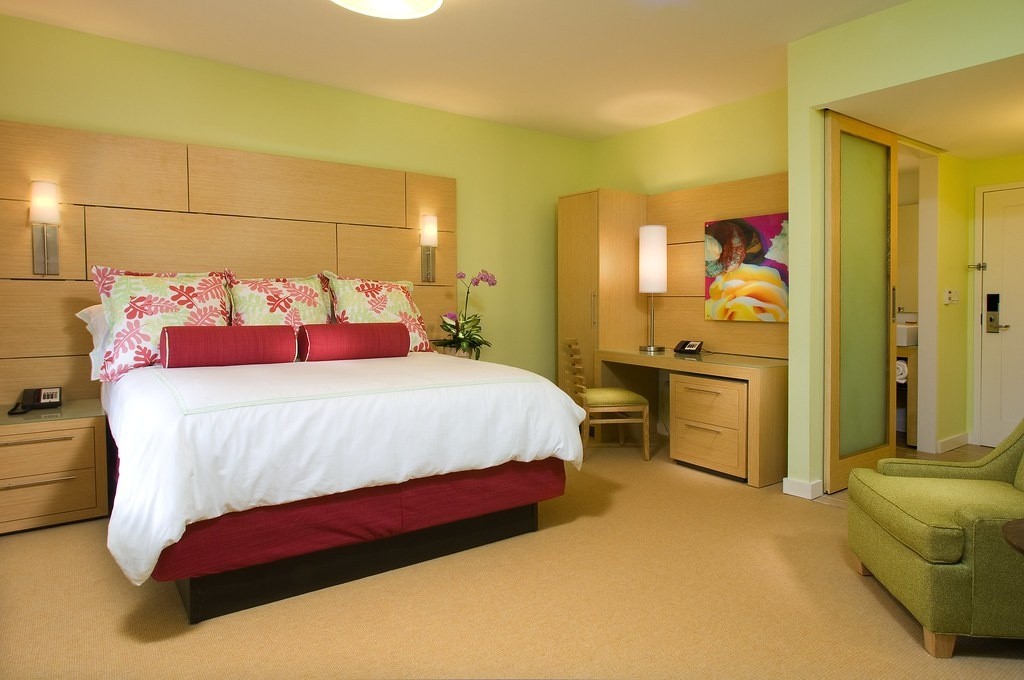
[559,338,650,462]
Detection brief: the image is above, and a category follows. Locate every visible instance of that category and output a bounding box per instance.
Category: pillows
[297,322,411,363]
[322,269,439,353]
[224,267,332,339]
[91,264,231,383]
[74,304,110,381]
[160,325,298,369]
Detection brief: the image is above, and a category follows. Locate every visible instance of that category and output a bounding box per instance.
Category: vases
[456,346,472,359]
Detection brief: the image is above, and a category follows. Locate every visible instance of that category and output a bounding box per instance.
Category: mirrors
[898,203,917,313]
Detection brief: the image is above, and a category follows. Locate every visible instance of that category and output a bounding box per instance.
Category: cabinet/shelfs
[669,373,748,482]
[557,188,647,441]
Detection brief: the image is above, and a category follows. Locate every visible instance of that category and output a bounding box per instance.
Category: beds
[0,119,587,626]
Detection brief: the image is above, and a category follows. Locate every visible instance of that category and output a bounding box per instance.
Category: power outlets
[664,375,670,390]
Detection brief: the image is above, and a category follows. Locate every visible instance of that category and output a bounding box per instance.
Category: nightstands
[0,398,108,534]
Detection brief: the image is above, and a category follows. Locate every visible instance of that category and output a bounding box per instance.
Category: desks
[594,348,788,488]
[897,346,917,447]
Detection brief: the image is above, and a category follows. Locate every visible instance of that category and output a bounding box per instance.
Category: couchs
[845,416,1024,658]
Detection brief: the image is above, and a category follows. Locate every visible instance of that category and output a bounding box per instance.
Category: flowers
[429,269,498,361]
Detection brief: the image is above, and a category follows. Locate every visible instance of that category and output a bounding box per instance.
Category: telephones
[674,340,703,354]
[21,386,62,410]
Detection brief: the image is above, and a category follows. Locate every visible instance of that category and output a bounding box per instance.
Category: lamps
[27,180,61,276]
[330,0,444,20]
[638,225,666,351]
[418,214,438,282]
